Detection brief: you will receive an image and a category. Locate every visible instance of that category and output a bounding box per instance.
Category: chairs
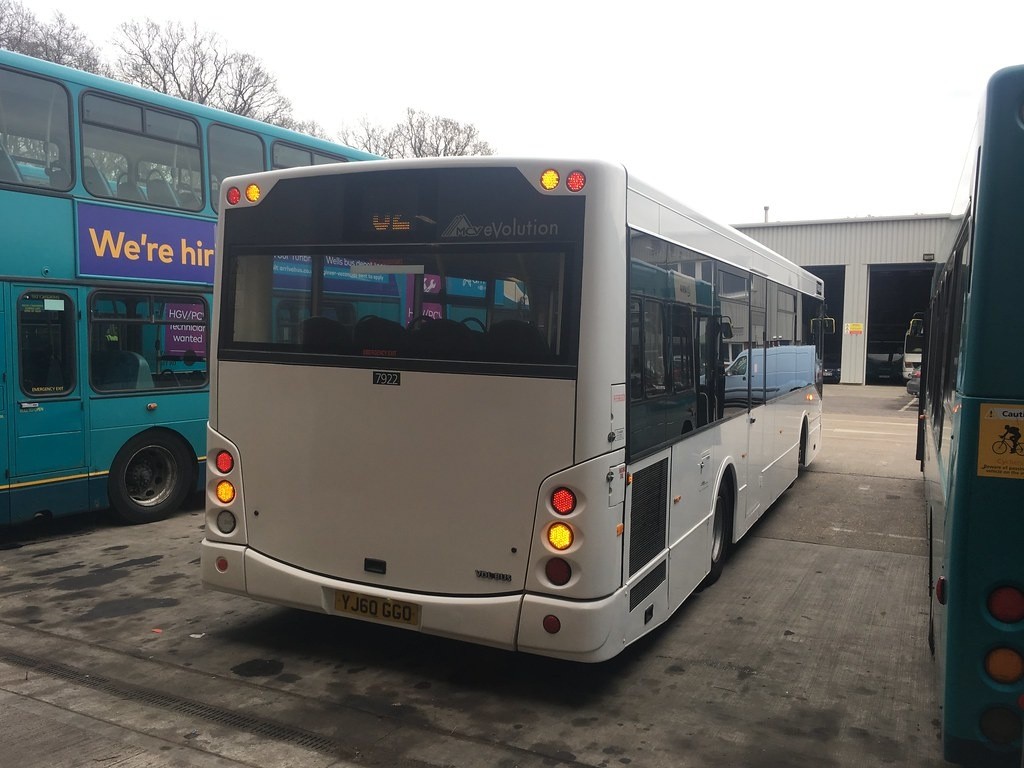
[296,313,549,360]
[0,144,219,210]
[90,350,204,390]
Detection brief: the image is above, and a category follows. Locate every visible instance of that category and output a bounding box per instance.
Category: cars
[907,365,922,397]
[823,353,841,384]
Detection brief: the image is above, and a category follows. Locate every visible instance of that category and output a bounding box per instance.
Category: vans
[700,344,816,405]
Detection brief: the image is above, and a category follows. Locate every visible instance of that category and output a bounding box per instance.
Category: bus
[901,318,924,380]
[629,255,722,357]
[197,158,835,663]
[904,64,1024,767]
[0,44,527,528]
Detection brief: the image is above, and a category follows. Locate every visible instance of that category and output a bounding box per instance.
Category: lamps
[923,254,934,261]
